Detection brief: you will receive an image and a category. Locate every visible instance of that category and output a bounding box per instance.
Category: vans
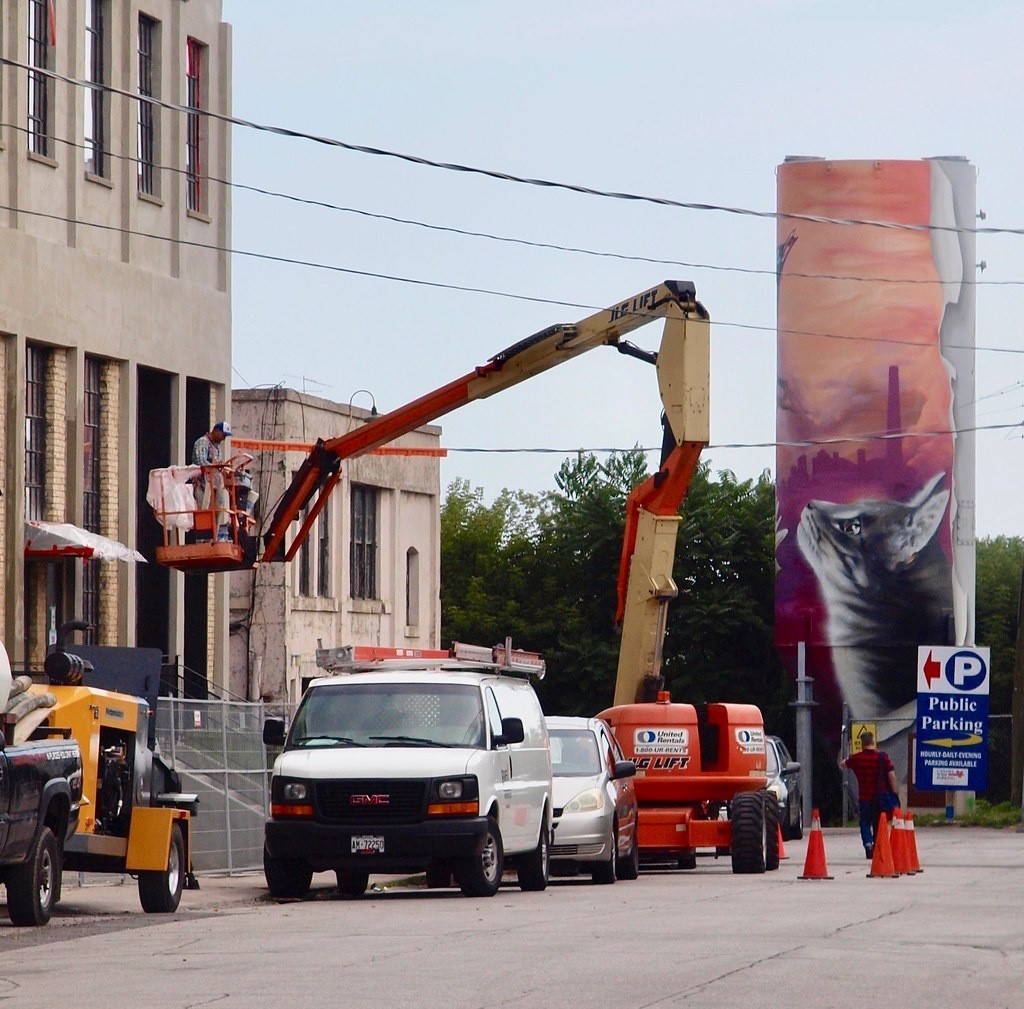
[259,645,557,901]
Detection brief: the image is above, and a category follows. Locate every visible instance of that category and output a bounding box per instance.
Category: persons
[193,421,233,542]
[840,732,898,859]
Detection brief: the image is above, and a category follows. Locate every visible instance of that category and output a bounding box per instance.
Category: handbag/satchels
[877,790,901,814]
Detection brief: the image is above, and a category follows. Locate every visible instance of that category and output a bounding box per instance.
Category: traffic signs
[910,645,987,793]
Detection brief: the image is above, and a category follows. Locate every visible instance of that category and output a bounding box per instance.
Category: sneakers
[866,845,874,858]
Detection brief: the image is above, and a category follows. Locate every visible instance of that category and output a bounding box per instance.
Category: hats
[215,421,232,436]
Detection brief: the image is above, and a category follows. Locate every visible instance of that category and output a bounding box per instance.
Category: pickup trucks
[0,718,89,929]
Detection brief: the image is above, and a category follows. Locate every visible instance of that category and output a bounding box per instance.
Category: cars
[542,713,642,884]
[710,735,806,842]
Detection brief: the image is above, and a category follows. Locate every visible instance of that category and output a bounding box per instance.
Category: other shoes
[218,525,234,543]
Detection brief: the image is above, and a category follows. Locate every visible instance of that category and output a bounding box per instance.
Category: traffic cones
[865,812,901,879]
[891,810,916,877]
[904,811,924,873]
[889,806,900,847]
[777,820,789,859]
[796,808,835,880]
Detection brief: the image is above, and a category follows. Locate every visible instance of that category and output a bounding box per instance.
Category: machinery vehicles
[152,278,792,878]
[26,619,202,913]
